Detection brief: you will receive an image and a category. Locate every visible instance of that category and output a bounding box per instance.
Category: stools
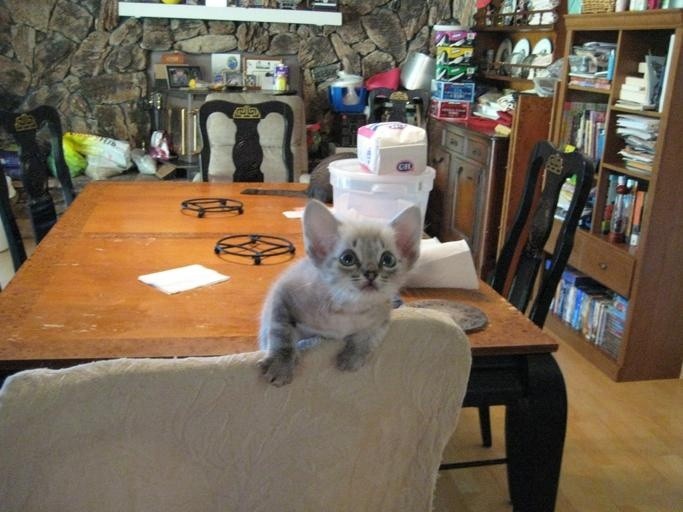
[328,86,367,114]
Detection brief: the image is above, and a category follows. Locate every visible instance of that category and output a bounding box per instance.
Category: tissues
[356,122,428,175]
[400,236,481,292]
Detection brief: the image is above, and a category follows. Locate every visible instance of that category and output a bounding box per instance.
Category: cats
[256,196,423,387]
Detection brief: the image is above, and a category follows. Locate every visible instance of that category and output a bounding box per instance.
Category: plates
[493,37,552,75]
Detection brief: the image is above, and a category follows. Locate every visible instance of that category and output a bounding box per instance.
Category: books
[542,50,666,367]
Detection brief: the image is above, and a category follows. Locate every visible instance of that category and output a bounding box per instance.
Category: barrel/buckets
[399,51,437,91]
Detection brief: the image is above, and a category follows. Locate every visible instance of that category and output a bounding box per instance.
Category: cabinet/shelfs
[523,7,683,382]
[470,0,566,82]
[426,115,511,280]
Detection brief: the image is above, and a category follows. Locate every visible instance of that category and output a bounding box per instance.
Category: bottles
[609,184,631,238]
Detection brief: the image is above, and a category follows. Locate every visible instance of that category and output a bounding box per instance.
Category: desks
[0,181,567,510]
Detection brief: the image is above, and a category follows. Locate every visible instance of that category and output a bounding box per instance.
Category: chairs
[1,105,77,273]
[198,99,295,183]
[479,140,594,448]
[0,308,473,511]
[197,92,310,183]
[369,87,431,125]
